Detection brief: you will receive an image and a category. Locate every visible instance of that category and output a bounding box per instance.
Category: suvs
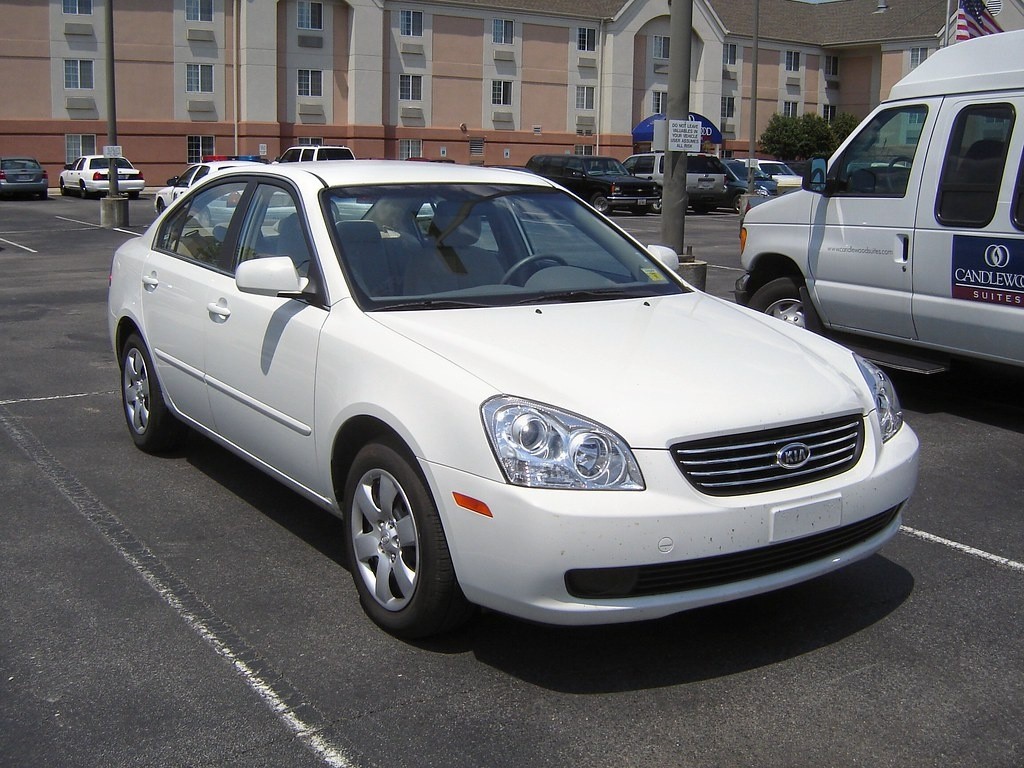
[526,154,663,215]
[715,158,803,214]
[272,144,356,165]
[621,150,729,214]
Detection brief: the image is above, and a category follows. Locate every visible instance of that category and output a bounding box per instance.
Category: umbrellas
[631,111,722,144]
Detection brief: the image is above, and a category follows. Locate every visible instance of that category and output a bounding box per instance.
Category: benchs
[170,220,420,299]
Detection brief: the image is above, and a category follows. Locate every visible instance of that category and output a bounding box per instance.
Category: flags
[956,0,1004,40]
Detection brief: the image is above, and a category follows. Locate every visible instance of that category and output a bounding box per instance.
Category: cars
[154,156,298,227]
[58,155,145,200]
[0,156,49,200]
[106,159,921,640]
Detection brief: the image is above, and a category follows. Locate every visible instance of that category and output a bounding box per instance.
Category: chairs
[591,166,602,170]
[956,139,1005,181]
[402,200,515,296]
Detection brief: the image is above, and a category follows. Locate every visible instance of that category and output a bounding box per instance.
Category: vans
[734,30,1024,374]
